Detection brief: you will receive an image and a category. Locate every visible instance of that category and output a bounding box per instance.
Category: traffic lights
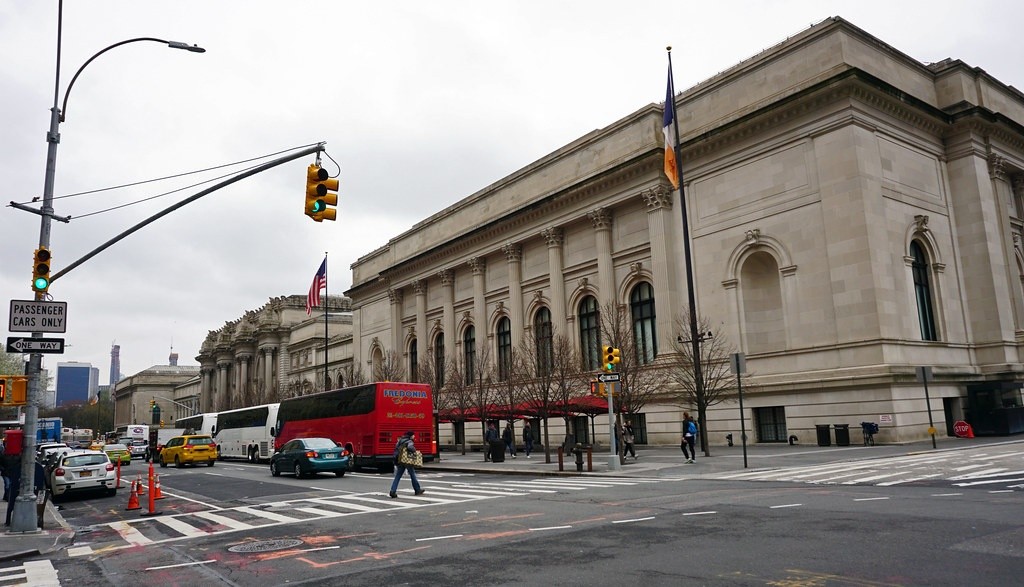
[160,421,165,428]
[603,345,615,372]
[312,176,339,223]
[152,400,158,409]
[590,378,605,395]
[304,163,329,216]
[31,245,53,292]
[0,379,7,402]
[612,348,621,365]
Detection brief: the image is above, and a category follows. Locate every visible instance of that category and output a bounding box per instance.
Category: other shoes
[690,460,696,463]
[415,489,425,495]
[526,456,531,459]
[390,492,397,498]
[683,458,691,464]
[634,454,638,459]
[512,454,516,458]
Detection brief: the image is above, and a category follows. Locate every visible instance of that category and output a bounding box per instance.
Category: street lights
[5,36,207,535]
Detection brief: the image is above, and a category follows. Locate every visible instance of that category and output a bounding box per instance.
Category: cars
[40,423,149,487]
[268,437,350,481]
[159,435,218,468]
[49,449,117,502]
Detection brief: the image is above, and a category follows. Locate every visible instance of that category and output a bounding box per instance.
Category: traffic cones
[136,473,145,495]
[154,474,167,500]
[125,480,144,510]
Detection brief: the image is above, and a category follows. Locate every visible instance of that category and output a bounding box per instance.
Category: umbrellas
[435,395,627,445]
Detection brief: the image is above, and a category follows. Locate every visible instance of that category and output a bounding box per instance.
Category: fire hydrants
[571,443,584,472]
[725,432,734,447]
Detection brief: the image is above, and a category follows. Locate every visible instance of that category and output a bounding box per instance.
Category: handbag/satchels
[397,446,422,468]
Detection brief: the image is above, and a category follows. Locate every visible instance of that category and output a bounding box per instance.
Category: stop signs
[952,420,970,439]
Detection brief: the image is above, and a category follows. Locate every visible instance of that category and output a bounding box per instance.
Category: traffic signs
[598,373,621,382]
[6,336,65,355]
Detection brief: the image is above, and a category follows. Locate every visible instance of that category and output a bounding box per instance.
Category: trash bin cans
[815,424,831,446]
[833,424,850,446]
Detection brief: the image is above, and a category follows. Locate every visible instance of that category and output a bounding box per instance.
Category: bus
[173,412,224,441]
[211,402,280,463]
[270,380,437,469]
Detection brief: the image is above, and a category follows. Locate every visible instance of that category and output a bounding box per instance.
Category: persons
[612,421,623,455]
[501,423,516,458]
[390,431,425,498]
[523,421,534,459]
[484,423,499,461]
[681,412,697,464]
[182,427,197,436]
[622,419,637,460]
[0,436,44,503]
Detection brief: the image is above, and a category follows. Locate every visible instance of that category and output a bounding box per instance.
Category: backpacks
[688,421,697,434]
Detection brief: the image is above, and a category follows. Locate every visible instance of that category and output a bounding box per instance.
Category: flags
[305,257,327,318]
[663,57,679,189]
[88,391,99,405]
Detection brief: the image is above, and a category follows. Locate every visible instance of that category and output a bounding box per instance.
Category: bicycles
[859,422,879,446]
